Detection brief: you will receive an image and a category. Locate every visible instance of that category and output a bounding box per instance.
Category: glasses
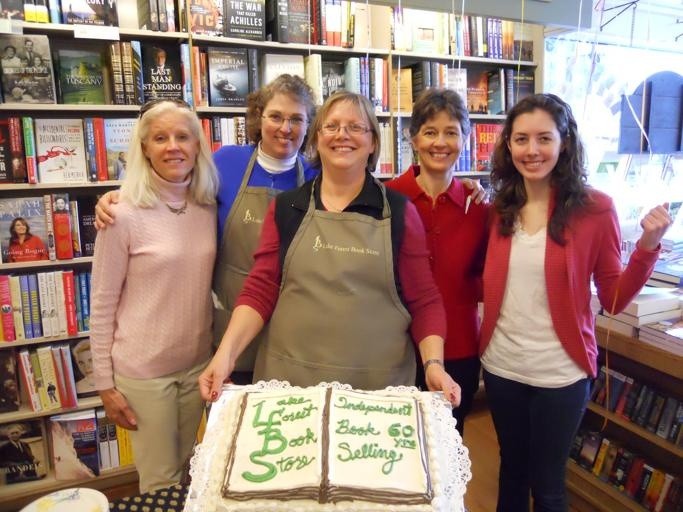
[317,121,371,135]
[139,98,192,117]
[260,108,310,127]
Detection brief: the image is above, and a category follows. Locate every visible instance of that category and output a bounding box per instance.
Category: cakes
[202,384,436,512]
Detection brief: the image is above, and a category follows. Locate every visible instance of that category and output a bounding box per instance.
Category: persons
[88,97,222,495]
[197,90,464,410]
[383,88,497,441]
[474,89,672,509]
[94,73,491,385]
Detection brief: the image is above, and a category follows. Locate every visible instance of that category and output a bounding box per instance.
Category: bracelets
[423,359,444,372]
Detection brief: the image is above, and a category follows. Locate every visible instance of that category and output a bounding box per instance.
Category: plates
[20,486,111,512]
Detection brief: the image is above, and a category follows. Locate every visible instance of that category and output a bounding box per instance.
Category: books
[0,192,134,489]
[1,0,535,184]
[568,230,682,512]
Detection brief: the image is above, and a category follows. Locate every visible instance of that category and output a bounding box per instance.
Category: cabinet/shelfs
[565,314,683,512]
[2,0,544,512]
